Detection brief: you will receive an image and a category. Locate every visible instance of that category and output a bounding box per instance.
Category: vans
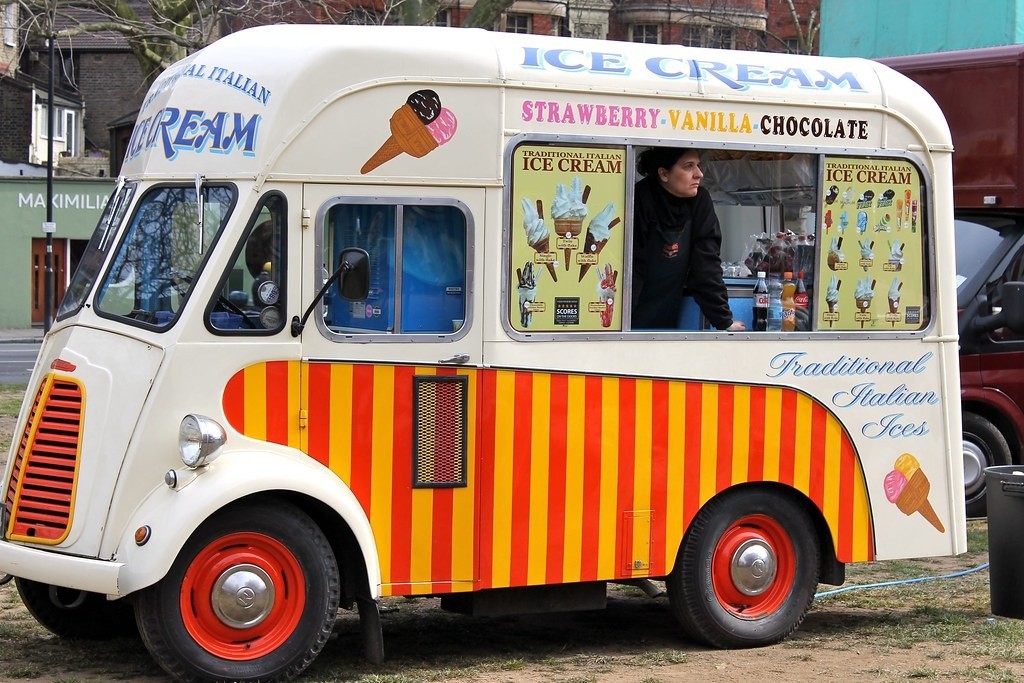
[867,43,1024,520]
[0,23,969,683]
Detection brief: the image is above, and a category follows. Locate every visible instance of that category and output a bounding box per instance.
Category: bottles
[793,272,809,331]
[753,272,768,331]
[766,273,783,331]
[797,235,808,245]
[772,233,784,247]
[782,273,796,330]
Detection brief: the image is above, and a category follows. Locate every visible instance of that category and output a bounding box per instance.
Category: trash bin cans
[983,464,1024,621]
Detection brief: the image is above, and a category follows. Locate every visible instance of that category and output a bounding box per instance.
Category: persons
[631,147,746,331]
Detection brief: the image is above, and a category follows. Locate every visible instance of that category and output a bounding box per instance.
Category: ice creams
[825,236,906,328]
[360,89,457,174]
[517,177,621,328]
[884,453,945,533]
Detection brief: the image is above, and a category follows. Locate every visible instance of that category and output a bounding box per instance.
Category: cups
[722,262,741,277]
